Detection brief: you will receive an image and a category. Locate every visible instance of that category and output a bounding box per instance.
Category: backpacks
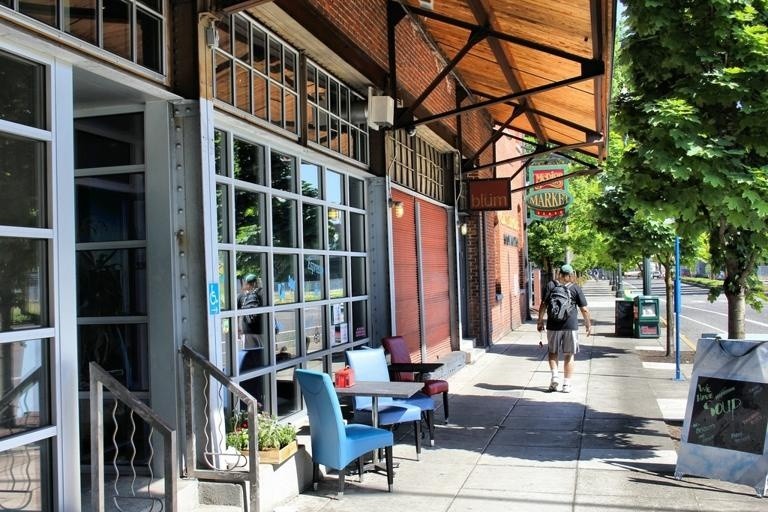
[241,287,263,334]
[546,279,577,325]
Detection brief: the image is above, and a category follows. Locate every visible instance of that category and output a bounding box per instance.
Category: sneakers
[548,376,560,391]
[562,382,572,393]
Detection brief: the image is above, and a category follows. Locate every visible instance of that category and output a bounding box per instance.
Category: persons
[535,264,592,393]
[238,274,279,349]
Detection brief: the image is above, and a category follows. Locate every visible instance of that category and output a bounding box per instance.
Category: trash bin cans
[633,296,661,339]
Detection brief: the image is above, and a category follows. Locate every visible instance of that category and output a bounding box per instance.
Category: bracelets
[536,319,545,324]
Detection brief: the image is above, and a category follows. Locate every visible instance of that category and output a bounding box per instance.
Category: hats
[558,264,574,274]
[244,274,258,284]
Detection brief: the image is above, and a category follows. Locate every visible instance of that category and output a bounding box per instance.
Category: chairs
[294,335,451,501]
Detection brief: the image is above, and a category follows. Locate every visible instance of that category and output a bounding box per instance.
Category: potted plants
[228,408,299,465]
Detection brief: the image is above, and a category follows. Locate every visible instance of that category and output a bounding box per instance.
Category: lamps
[368,84,396,131]
